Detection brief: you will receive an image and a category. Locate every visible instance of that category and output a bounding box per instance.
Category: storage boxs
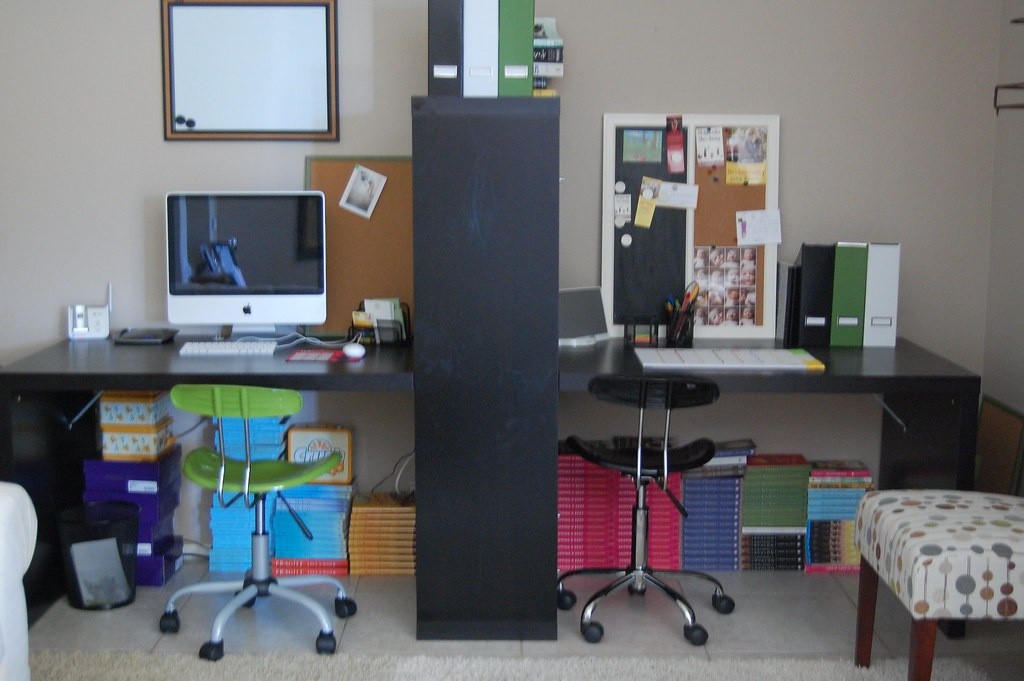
[97,390,177,463]
[83,444,184,587]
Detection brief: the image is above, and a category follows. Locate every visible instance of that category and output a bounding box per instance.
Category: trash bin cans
[55,500,140,610]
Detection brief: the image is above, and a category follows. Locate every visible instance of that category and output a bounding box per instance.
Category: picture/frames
[297,197,319,259]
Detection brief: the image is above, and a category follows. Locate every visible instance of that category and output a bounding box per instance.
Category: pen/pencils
[663,281,702,340]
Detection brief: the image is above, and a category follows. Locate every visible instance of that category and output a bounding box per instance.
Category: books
[557,441,874,574]
[208,416,416,575]
[777,260,800,345]
[532,16,564,97]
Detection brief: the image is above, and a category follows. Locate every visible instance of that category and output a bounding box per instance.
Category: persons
[723,126,761,162]
[672,118,678,136]
[694,247,756,326]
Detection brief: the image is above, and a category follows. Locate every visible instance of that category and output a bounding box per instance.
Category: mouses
[343,343,365,359]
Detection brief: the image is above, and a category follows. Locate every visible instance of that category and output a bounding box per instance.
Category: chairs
[160,383,356,661]
[556,374,735,645]
[855,488,1024,681]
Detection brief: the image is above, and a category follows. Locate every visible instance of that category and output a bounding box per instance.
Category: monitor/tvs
[166,191,327,342]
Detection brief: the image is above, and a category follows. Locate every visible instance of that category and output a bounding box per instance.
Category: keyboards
[180,341,278,357]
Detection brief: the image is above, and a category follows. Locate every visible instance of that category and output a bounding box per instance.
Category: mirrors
[161,0,340,142]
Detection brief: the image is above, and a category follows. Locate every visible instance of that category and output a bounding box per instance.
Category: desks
[0,338,415,629]
[557,337,980,640]
